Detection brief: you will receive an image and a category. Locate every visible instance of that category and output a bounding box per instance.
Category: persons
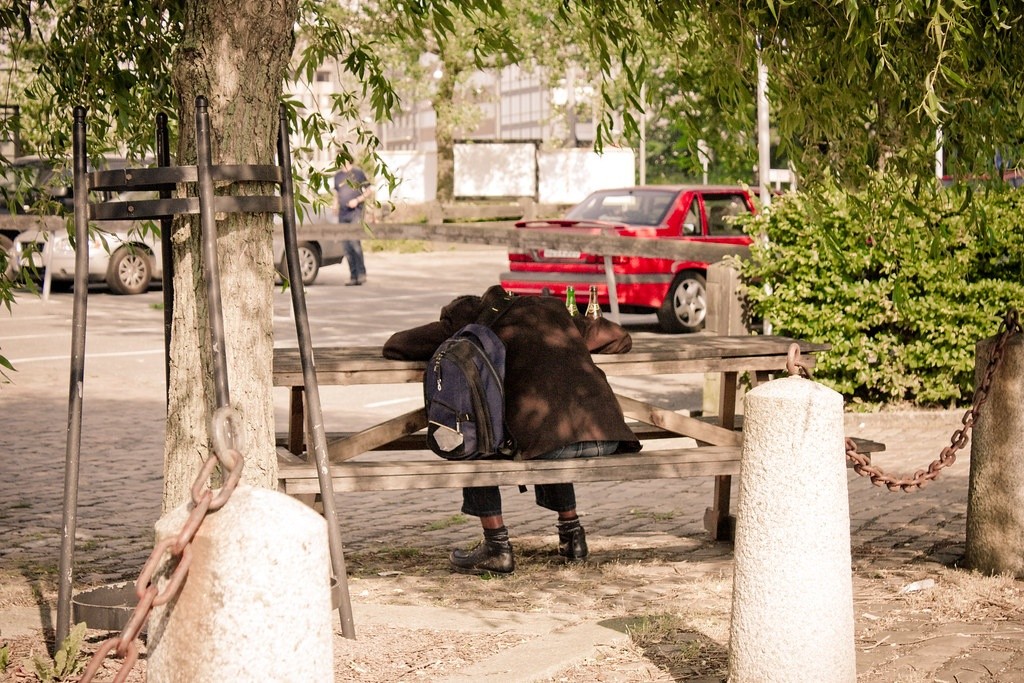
[382,284,643,575]
[331,155,373,286]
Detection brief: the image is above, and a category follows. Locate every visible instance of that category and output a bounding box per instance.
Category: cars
[500,186,784,334]
[939,169,1024,190]
[12,187,346,297]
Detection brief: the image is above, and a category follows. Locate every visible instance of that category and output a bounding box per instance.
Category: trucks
[0,152,180,278]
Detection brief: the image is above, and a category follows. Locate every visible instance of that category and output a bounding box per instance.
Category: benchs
[276,444,884,496]
[274,414,745,451]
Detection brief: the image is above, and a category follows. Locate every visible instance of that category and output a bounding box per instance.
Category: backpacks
[424,296,522,460]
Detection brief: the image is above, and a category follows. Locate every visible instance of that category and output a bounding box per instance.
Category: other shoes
[357,274,367,284]
[345,278,357,285]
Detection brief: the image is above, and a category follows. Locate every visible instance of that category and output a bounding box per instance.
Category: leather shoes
[535,525,588,562]
[449,530,515,576]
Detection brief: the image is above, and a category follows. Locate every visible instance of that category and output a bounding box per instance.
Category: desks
[273,335,833,541]
